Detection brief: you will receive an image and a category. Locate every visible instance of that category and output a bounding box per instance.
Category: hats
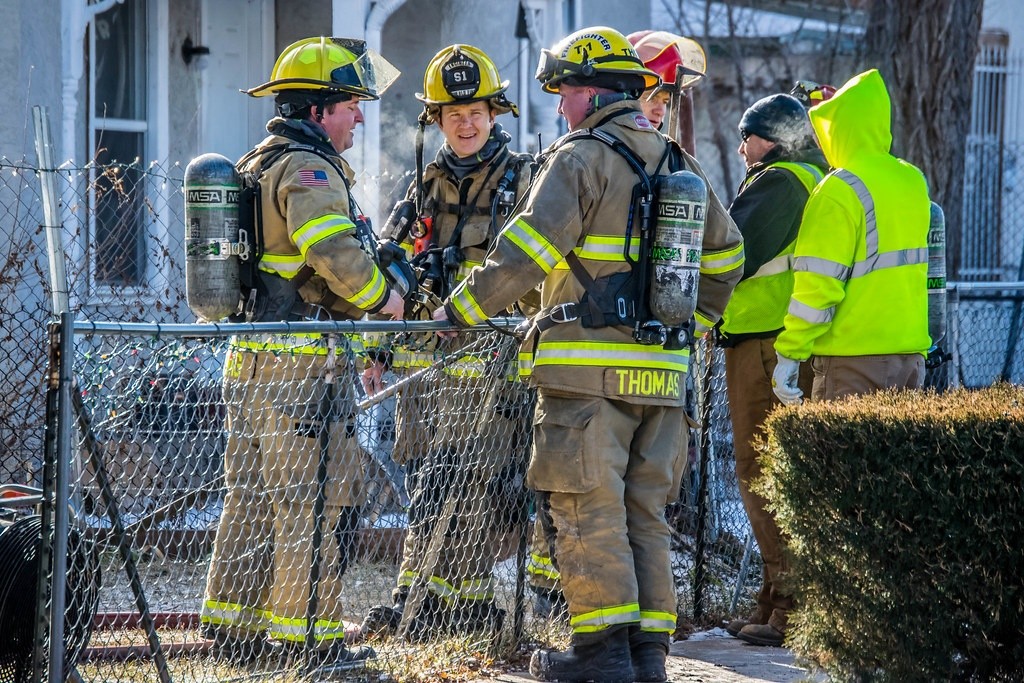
[738,93,811,149]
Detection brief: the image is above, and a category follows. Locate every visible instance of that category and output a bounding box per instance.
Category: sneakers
[630,641,668,683]
[453,597,508,631]
[364,591,451,637]
[309,643,377,668]
[530,626,634,683]
[215,633,280,666]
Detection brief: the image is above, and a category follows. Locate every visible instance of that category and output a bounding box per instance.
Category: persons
[196,33,405,671]
[360,25,929,679]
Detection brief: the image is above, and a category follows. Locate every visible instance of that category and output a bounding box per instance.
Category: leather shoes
[738,623,786,645]
[727,618,761,637]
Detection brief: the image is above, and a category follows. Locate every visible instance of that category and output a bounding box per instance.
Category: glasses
[741,130,752,143]
[534,49,579,81]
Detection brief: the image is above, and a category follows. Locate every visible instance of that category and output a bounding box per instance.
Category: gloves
[771,350,806,406]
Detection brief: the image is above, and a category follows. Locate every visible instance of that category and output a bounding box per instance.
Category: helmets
[624,30,685,91]
[535,26,663,94]
[248,37,380,102]
[806,84,838,108]
[415,44,509,106]
